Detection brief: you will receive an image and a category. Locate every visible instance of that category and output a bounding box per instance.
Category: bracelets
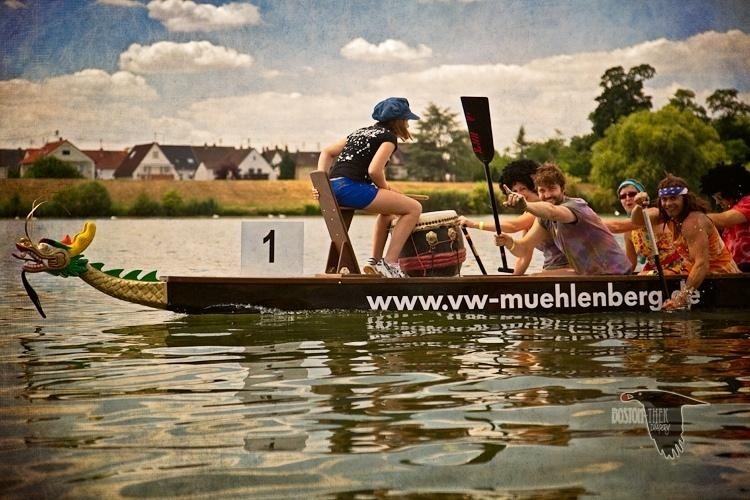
[509,236,517,252]
[478,220,484,232]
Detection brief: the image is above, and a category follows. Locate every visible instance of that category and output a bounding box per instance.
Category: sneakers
[364,257,411,278]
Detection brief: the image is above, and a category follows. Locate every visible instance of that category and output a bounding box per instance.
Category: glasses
[619,192,637,199]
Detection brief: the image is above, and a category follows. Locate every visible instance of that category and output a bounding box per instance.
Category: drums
[390,209,466,278]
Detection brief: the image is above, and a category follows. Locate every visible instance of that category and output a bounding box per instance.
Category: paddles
[461,97,514,273]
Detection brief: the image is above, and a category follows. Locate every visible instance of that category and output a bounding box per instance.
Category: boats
[11,197,745,313]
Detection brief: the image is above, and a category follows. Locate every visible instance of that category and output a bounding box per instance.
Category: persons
[492,161,634,275]
[598,176,685,277]
[629,170,742,314]
[236,318,744,465]
[310,96,428,279]
[453,156,569,277]
[697,163,750,274]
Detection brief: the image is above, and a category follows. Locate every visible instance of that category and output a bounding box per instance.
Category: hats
[372,97,419,122]
